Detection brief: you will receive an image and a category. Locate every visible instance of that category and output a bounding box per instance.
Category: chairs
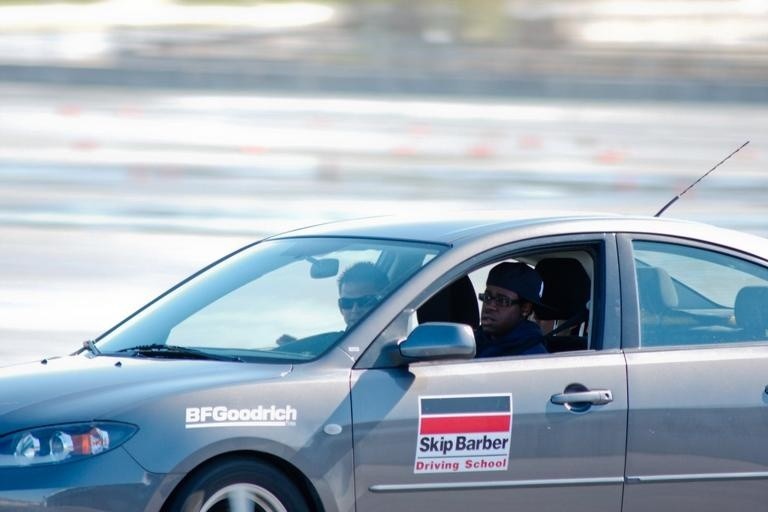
[416,255,768,350]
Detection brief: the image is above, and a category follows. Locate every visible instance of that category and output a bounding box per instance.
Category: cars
[0,213,768,510]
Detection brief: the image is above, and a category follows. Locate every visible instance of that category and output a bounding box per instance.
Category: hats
[486,261,544,305]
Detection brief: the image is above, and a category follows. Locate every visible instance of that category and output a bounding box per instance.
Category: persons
[272,259,392,348]
[532,257,591,352]
[472,261,548,361]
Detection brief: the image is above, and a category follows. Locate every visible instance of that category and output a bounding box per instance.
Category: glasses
[479,293,527,307]
[338,294,381,309]
